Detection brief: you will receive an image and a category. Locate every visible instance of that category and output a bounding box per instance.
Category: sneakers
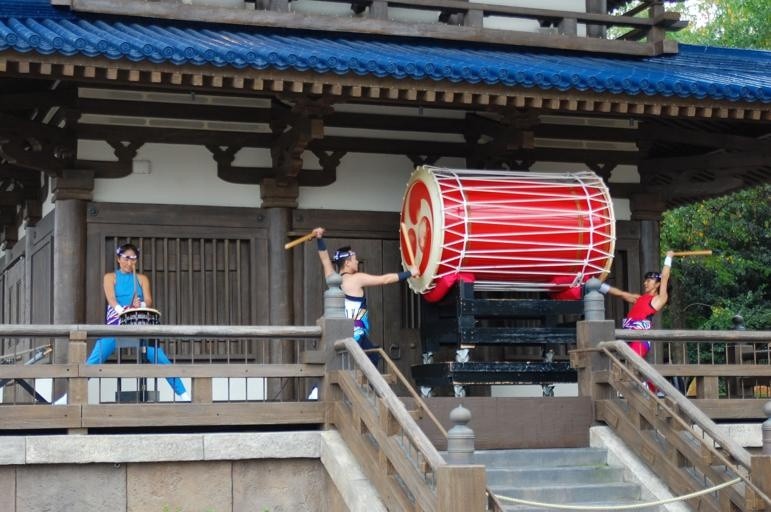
[309,387,319,401]
[52,392,68,408]
[178,391,190,400]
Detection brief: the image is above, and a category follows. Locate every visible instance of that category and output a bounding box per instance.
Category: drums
[399,165,616,295]
[118,309,162,325]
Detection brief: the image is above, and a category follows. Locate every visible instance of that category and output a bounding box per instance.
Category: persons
[308,229,420,401]
[52,243,193,405]
[590,252,672,397]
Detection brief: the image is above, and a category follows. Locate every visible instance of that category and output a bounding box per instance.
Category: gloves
[585,276,603,293]
